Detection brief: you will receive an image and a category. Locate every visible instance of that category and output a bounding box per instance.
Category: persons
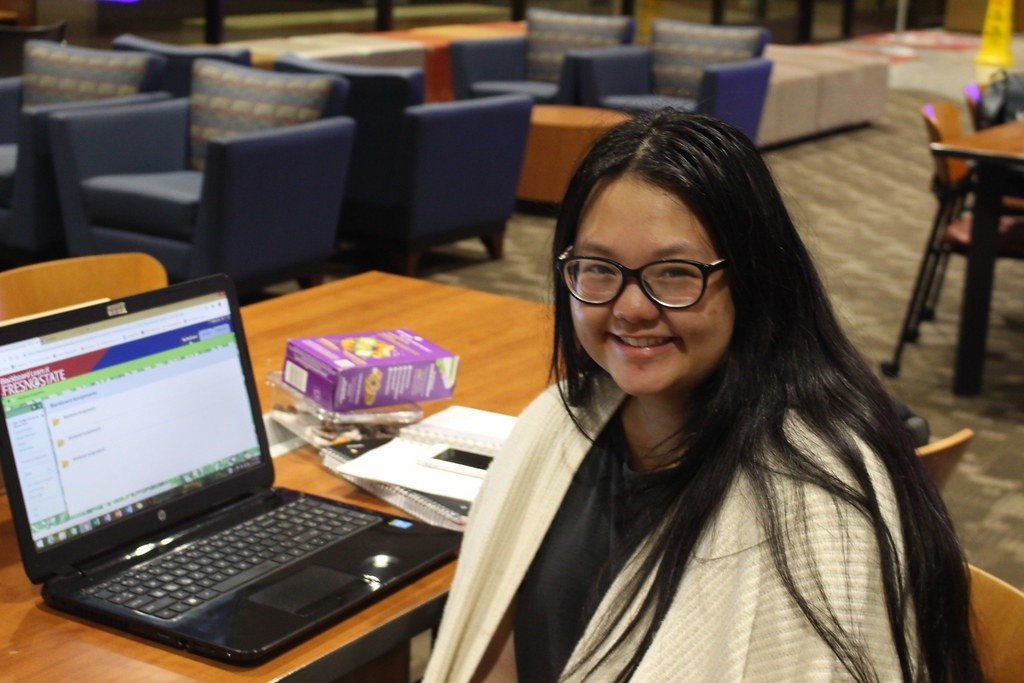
[422,106,986,683]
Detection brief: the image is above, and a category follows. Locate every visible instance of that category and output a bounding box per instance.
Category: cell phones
[415,444,495,478]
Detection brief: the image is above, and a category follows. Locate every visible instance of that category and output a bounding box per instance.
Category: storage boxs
[279,328,460,409]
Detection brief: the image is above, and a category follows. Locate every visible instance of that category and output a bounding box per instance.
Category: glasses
[555,245,726,309]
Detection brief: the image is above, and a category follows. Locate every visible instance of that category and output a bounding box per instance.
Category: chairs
[0,252,168,320]
[915,428,1024,682]
[881,70,1024,378]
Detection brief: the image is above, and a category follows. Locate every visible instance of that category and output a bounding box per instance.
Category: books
[322,437,471,531]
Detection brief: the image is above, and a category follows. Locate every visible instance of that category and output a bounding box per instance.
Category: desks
[1,268,643,683]
[927,119,1023,398]
[515,103,634,215]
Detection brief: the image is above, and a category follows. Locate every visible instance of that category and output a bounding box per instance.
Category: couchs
[0,0,891,307]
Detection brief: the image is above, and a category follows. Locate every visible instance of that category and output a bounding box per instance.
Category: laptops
[0,273,464,668]
